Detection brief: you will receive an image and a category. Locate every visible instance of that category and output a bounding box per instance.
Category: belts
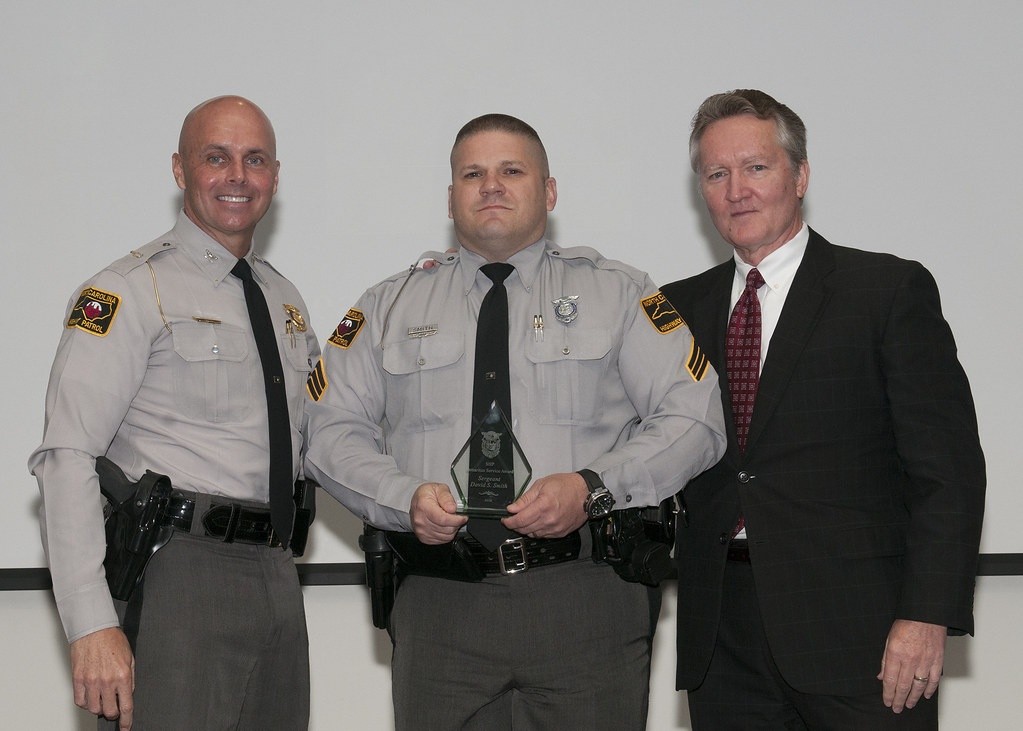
[155,497,292,548]
[728,540,750,562]
[393,519,604,582]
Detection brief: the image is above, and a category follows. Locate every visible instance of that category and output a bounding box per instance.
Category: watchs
[576,469,616,520]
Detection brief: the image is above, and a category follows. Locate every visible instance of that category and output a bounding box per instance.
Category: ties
[229,257,293,552]
[467,262,516,553]
[726,268,766,541]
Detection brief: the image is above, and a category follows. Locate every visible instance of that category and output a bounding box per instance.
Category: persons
[659,89,987,731]
[27,95,321,731]
[304,114,727,730]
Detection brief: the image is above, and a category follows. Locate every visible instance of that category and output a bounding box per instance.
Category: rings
[914,675,928,682]
